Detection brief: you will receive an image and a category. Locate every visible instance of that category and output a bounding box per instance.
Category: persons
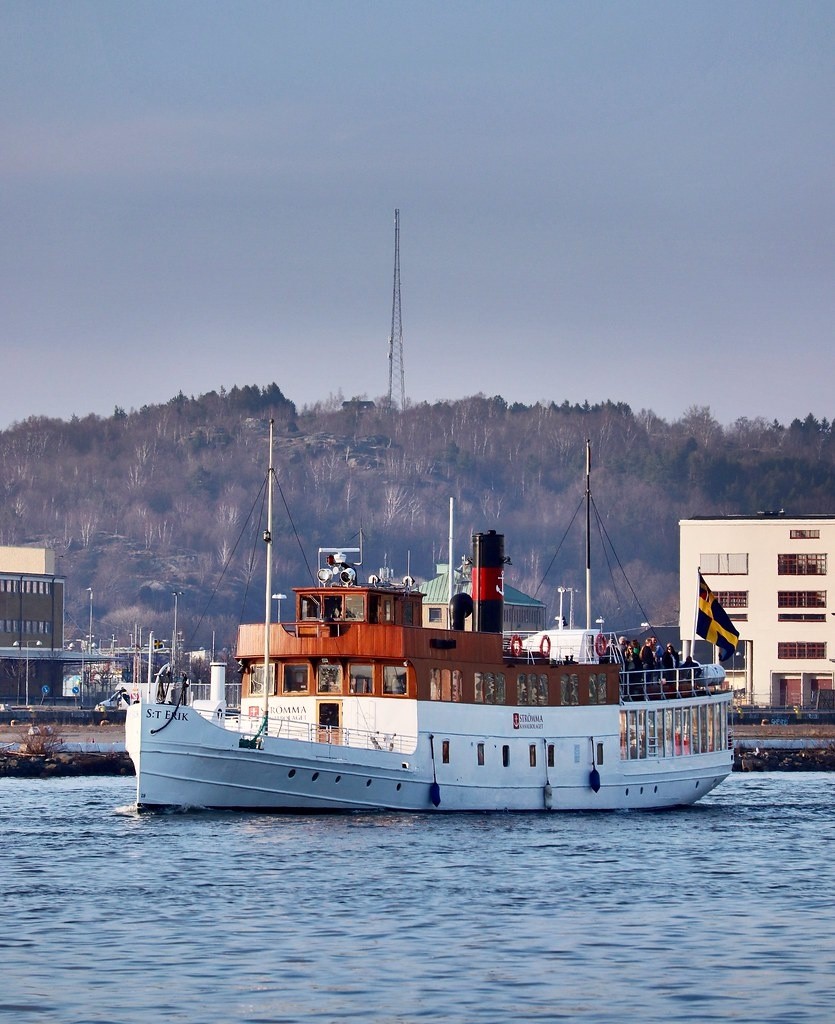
[606,636,702,702]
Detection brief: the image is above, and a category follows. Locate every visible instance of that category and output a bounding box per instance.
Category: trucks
[93,681,181,712]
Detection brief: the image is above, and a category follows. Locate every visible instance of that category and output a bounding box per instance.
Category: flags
[153,639,164,651]
[695,572,740,661]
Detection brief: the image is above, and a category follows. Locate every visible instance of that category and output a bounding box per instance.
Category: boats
[125,420,741,818]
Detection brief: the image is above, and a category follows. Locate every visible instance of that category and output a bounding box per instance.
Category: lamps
[262,529,272,543]
[317,568,333,588]
[340,568,355,587]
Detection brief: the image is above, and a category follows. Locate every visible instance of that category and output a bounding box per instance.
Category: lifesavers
[510,634,523,657]
[539,634,551,657]
[595,634,607,657]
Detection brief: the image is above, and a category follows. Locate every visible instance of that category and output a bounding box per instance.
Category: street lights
[13,639,43,706]
[86,588,97,658]
[171,591,185,676]
